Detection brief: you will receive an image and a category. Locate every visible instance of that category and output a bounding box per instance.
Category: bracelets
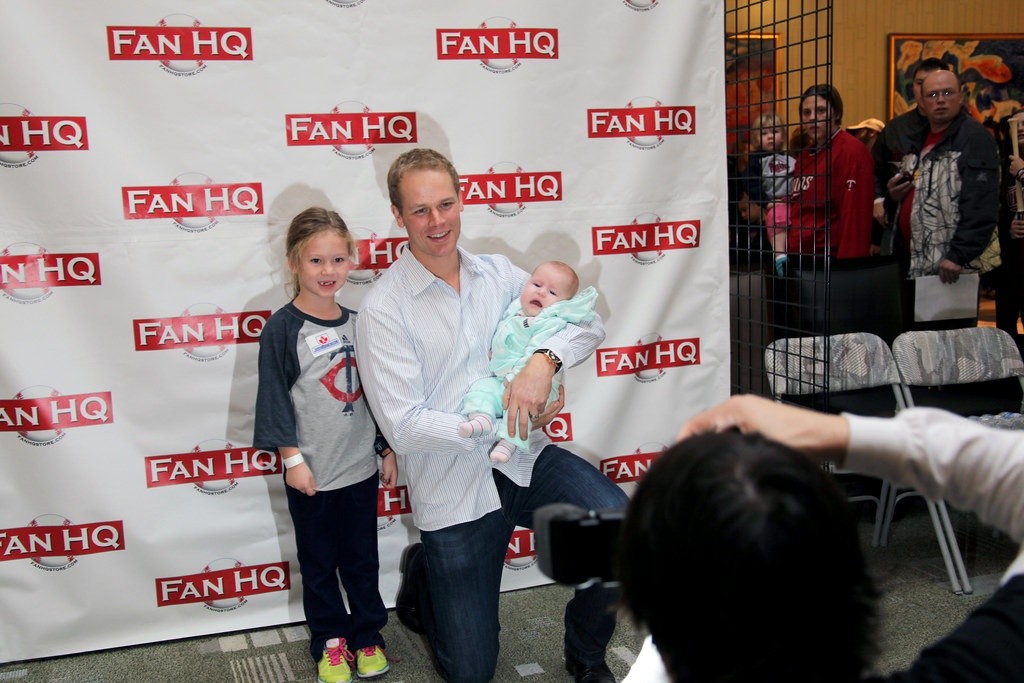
[373,435,394,458]
[283,452,305,470]
[1015,167,1024,181]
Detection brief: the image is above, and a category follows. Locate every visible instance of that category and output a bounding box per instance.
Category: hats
[845,118,885,134]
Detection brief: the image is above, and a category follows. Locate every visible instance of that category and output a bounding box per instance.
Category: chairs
[881,327,1024,594]
[764,332,963,594]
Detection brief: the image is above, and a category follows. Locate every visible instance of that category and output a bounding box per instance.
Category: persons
[981,109,1024,353]
[882,69,1002,333]
[619,391,1023,683]
[253,207,400,683]
[352,147,632,683]
[785,83,875,335]
[869,58,950,266]
[736,111,886,276]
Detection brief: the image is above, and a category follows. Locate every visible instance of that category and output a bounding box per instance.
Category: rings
[530,414,540,421]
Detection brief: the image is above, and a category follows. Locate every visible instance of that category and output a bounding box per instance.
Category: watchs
[534,349,563,374]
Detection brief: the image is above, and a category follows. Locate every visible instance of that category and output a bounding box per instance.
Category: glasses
[923,86,959,102]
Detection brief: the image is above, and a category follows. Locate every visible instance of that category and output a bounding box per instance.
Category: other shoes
[775,258,785,277]
[565,654,616,683]
[395,543,420,635]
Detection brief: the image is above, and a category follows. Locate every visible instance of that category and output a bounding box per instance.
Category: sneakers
[350,645,400,678]
[317,638,352,683]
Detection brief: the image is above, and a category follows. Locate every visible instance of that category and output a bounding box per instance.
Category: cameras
[533,502,628,593]
[895,153,919,186]
